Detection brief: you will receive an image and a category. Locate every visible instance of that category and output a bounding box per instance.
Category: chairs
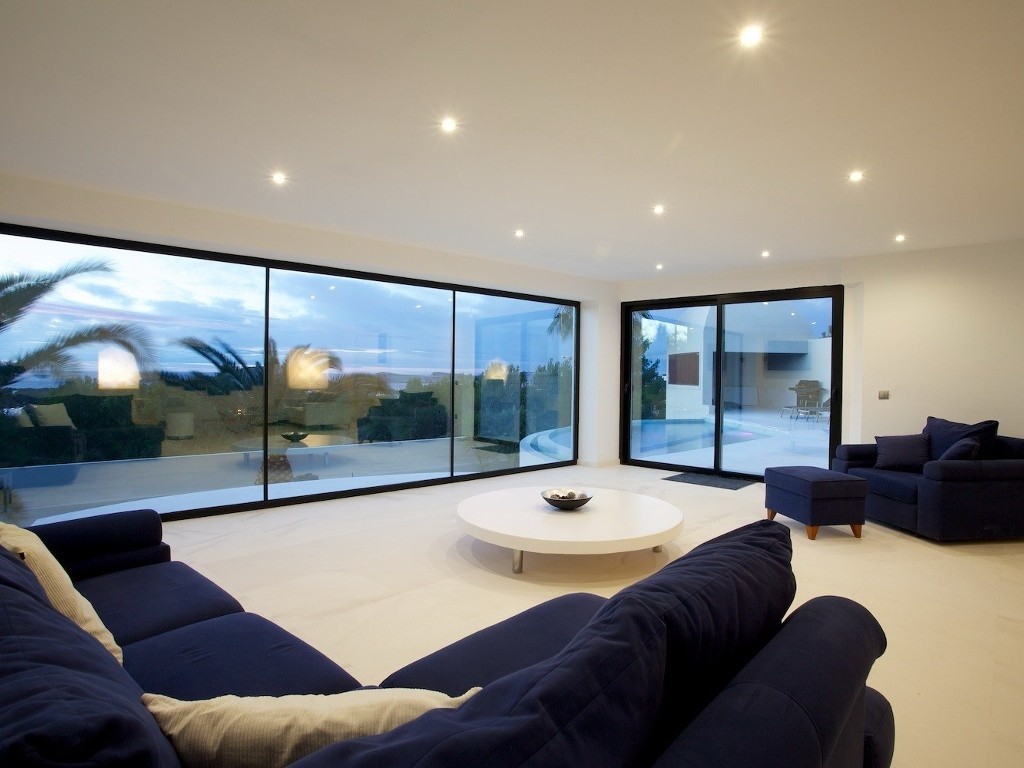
[781,398,830,423]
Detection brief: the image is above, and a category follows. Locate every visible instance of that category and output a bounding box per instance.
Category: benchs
[765,466,867,541]
[289,402,356,430]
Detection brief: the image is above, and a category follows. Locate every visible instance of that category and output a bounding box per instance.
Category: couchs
[0,393,165,467]
[832,416,1024,542]
[0,509,895,767]
[357,404,447,443]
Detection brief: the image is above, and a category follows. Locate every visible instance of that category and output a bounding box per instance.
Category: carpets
[662,473,757,491]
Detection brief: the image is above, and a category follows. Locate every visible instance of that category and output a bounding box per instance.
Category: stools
[166,412,194,439]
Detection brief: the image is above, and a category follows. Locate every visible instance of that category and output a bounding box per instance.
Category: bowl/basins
[280,431,308,443]
[541,489,592,510]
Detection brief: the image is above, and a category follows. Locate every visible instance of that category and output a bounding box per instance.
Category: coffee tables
[457,486,684,575]
[233,435,355,451]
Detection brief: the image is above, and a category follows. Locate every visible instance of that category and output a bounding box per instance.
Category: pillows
[4,406,35,428]
[378,390,438,417]
[0,522,122,667]
[931,420,999,460]
[30,403,77,430]
[142,687,484,768]
[874,434,931,470]
